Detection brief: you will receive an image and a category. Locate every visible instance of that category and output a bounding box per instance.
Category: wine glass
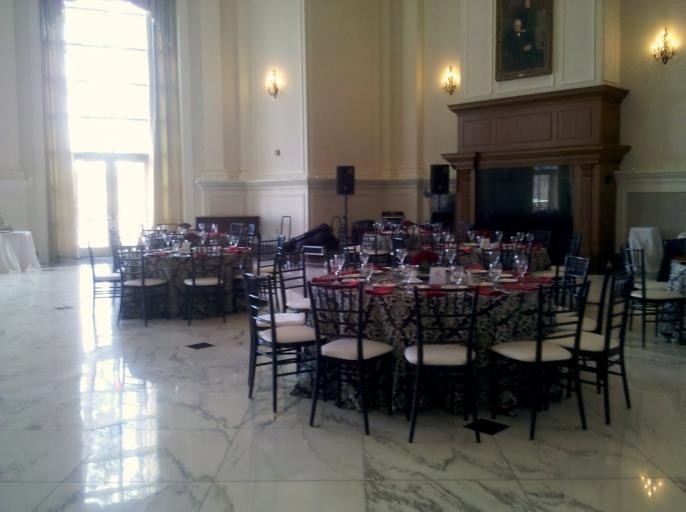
[442,231,451,248]
[510,236,519,251]
[514,254,530,288]
[517,232,525,247]
[444,245,458,264]
[467,231,476,243]
[358,251,369,266]
[488,262,503,290]
[525,233,534,248]
[372,219,443,236]
[432,232,442,250]
[395,248,408,266]
[147,223,240,252]
[333,254,346,268]
[329,259,342,285]
[494,231,503,244]
[403,264,419,293]
[361,262,374,291]
[446,257,466,290]
[485,244,501,266]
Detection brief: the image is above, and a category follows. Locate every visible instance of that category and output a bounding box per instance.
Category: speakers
[431,211,451,233]
[658,240,686,280]
[336,166,354,194]
[352,220,374,244]
[431,164,449,194]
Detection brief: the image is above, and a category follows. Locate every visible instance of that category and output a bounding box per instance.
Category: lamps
[651,27,676,66]
[441,64,458,97]
[266,67,279,100]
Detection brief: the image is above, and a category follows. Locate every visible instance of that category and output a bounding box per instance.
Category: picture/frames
[494,1,555,84]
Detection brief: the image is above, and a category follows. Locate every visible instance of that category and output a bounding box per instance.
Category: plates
[470,269,488,274]
[399,284,432,291]
[382,267,392,269]
[342,278,366,283]
[342,274,361,277]
[497,278,520,283]
[372,269,383,274]
[498,273,513,278]
[372,283,398,288]
[441,285,469,290]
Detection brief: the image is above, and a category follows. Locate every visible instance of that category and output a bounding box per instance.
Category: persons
[521,0,538,44]
[509,18,542,68]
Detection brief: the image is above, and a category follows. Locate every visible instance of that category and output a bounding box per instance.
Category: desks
[1,229,42,276]
